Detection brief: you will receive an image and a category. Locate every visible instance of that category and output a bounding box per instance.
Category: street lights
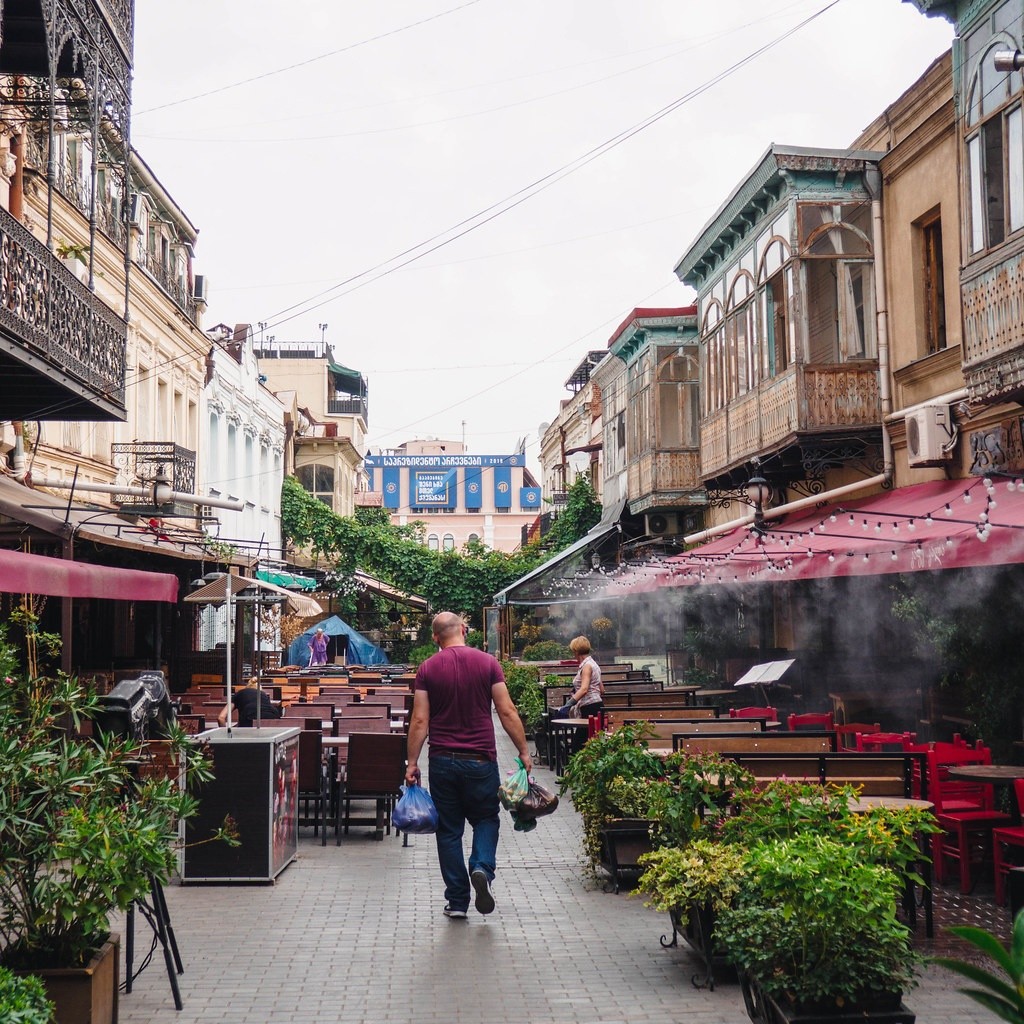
[590,550,601,658]
[744,454,774,707]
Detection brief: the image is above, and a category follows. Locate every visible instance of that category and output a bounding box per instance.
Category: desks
[550,718,589,778]
[663,685,702,692]
[765,721,782,730]
[828,688,924,748]
[321,736,350,748]
[786,796,935,939]
[320,691,414,697]
[948,764,1024,826]
[201,700,282,707]
[691,765,732,814]
[205,721,239,729]
[941,712,977,726]
[334,708,409,721]
[690,689,738,707]
[640,746,677,761]
[321,720,404,734]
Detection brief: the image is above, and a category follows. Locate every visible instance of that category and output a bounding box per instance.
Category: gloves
[559,697,578,715]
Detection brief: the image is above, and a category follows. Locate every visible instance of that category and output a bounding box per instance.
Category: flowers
[0,605,245,967]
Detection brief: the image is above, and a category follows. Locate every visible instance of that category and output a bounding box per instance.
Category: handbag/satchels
[391,783,438,834]
[498,757,559,833]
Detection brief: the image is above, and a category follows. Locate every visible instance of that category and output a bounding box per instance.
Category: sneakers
[443,903,467,919]
[471,866,496,915]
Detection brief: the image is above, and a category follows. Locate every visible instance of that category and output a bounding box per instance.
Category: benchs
[526,659,936,944]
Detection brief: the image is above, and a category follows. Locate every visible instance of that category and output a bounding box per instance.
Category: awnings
[606,474,1024,597]
[0,475,431,746]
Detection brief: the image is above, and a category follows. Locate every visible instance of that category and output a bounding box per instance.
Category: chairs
[991,778,1024,905]
[928,747,1011,894]
[726,707,984,842]
[588,711,625,739]
[560,660,581,665]
[46,660,420,848]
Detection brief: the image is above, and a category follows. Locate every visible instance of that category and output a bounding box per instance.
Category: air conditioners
[191,273,210,306]
[645,511,678,536]
[121,191,150,235]
[903,403,960,470]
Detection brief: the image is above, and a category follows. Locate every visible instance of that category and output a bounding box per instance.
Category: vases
[0,930,119,1024]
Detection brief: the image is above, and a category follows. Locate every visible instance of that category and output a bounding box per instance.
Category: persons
[307,628,330,666]
[218,677,279,728]
[554,636,604,784]
[405,612,532,919]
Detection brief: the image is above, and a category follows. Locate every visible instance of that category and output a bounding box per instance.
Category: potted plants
[495,660,566,768]
[56,237,105,288]
[557,720,671,897]
[711,830,918,1024]
[624,837,752,993]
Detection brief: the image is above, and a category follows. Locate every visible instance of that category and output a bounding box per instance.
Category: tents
[281,613,389,667]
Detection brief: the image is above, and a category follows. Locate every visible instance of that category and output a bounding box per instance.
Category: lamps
[591,551,601,569]
[739,461,774,535]
